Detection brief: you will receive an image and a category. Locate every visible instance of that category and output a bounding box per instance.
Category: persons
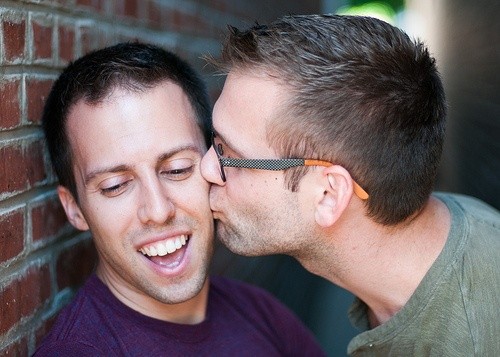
[200,14,500,357]
[30,44,330,357]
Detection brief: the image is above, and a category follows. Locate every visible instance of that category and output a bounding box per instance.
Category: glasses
[212,130,369,200]
